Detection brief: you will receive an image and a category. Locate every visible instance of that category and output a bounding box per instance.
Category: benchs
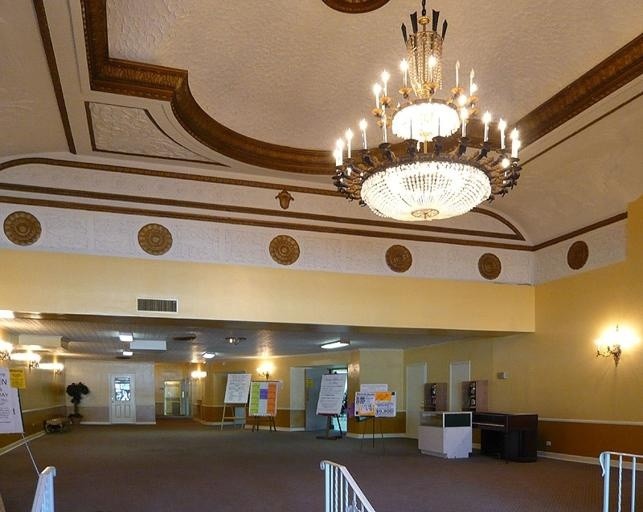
[45,416,71,433]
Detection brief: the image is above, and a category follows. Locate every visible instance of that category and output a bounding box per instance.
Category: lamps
[595,324,640,367]
[332,0,522,222]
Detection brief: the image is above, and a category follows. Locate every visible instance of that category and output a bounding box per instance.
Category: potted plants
[67,382,89,424]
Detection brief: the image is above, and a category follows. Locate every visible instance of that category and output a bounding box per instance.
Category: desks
[472,411,539,463]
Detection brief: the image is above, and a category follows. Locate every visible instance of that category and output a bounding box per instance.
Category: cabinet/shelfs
[417,410,472,460]
[462,380,488,412]
[423,382,447,411]
